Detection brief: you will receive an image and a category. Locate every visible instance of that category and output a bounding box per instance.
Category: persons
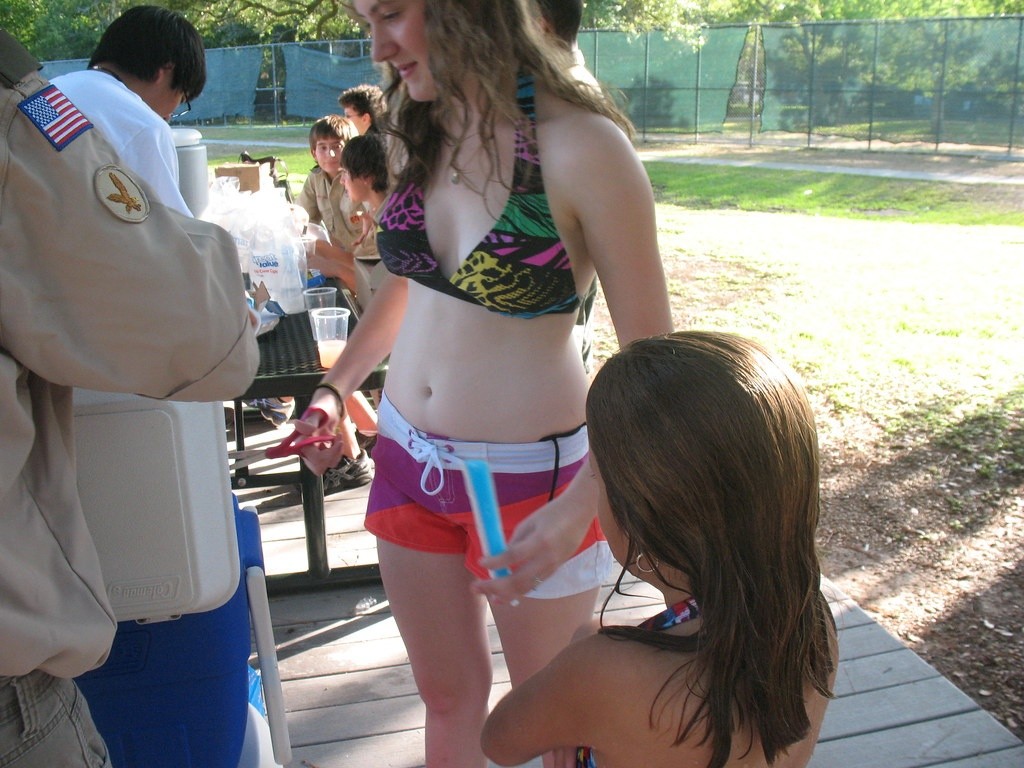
[241,85,395,498]
[0,27,260,768]
[481,331,838,768]
[528,0,603,378]
[46,6,207,218]
[293,0,674,768]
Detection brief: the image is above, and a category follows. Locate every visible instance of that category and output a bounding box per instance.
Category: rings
[532,574,542,585]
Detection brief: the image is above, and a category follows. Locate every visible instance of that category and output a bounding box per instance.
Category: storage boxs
[213,163,270,193]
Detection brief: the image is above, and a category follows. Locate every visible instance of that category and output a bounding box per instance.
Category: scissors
[227,406,337,471]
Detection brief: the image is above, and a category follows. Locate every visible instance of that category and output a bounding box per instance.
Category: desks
[220,273,392,596]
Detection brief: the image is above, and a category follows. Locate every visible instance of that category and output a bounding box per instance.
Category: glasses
[172,86,191,118]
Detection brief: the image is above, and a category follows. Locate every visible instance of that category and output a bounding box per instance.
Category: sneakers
[244,396,295,427]
[355,428,377,458]
[321,446,375,495]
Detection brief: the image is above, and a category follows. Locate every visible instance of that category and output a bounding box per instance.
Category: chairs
[69,493,290,768]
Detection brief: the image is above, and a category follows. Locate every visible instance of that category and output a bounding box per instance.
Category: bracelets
[312,383,344,418]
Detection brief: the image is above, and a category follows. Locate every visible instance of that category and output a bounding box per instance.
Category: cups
[312,307,350,369]
[302,237,317,256]
[303,287,337,341]
